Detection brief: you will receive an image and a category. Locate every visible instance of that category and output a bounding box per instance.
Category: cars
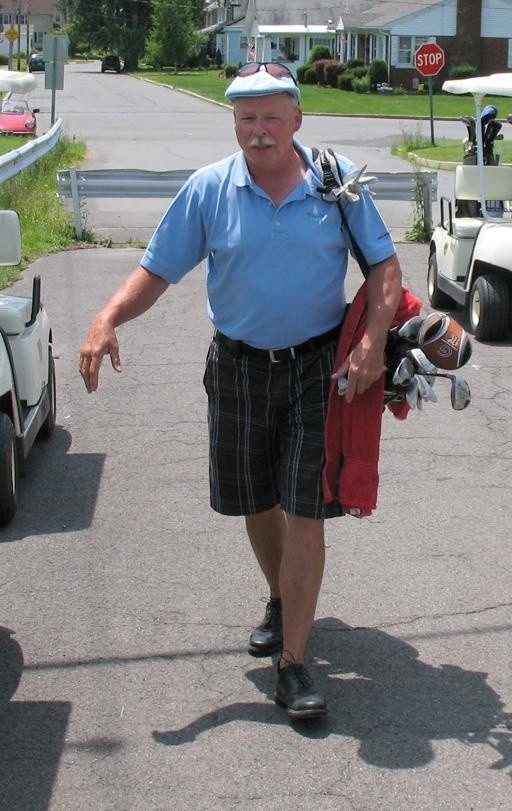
[0,98,41,136]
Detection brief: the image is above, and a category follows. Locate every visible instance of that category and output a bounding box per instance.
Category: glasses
[236,60,298,84]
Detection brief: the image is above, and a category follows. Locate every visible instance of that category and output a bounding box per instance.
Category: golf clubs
[459,105,503,154]
[387,311,472,410]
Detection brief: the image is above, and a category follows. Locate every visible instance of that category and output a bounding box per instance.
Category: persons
[80,62,402,720]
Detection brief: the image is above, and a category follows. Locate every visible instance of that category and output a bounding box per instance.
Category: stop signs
[414,43,446,76]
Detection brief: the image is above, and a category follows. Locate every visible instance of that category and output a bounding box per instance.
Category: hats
[226,64,300,101]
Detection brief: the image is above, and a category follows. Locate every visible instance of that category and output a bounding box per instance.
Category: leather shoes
[250,597,283,656]
[275,649,326,718]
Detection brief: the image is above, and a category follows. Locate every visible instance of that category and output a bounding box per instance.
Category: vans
[99,55,121,73]
[28,52,45,73]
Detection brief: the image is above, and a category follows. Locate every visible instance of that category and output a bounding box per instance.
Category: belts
[211,329,341,364]
